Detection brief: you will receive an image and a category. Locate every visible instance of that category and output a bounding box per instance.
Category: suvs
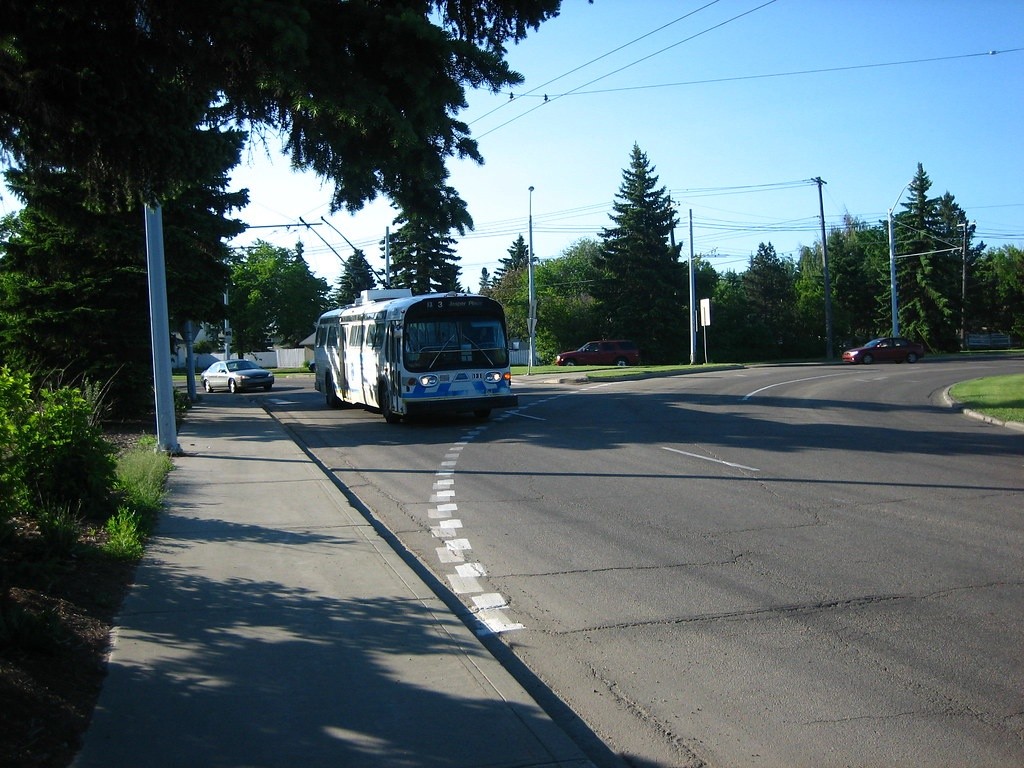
[555,340,640,366]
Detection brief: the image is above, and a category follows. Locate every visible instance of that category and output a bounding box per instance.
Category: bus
[298,215,519,431]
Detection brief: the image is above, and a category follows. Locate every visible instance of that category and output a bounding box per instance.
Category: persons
[470,327,493,348]
[882,341,888,347]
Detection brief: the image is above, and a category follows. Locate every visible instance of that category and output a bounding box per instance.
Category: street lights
[887,179,917,338]
[528,187,535,366]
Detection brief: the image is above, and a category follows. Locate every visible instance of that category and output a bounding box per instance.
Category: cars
[842,337,923,364]
[200,359,275,394]
